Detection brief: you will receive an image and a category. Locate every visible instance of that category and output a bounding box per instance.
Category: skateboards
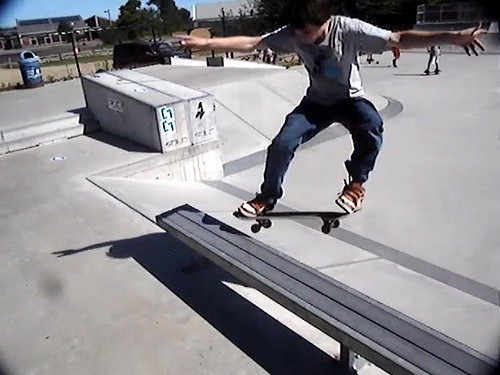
[423,70,442,74]
[233,208,361,234]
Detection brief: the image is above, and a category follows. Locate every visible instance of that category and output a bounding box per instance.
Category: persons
[423,45,441,72]
[170,0,489,219]
[263,47,276,64]
[367,52,374,61]
[391,47,401,68]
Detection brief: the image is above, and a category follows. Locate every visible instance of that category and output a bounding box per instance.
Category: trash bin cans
[18,50,43,88]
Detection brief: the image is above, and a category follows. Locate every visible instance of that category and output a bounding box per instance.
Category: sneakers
[238,190,277,218]
[335,178,365,214]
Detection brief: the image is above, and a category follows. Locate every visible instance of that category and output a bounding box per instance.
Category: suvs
[114,43,190,72]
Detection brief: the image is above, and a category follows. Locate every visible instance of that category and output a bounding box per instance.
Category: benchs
[156,203,500,375]
